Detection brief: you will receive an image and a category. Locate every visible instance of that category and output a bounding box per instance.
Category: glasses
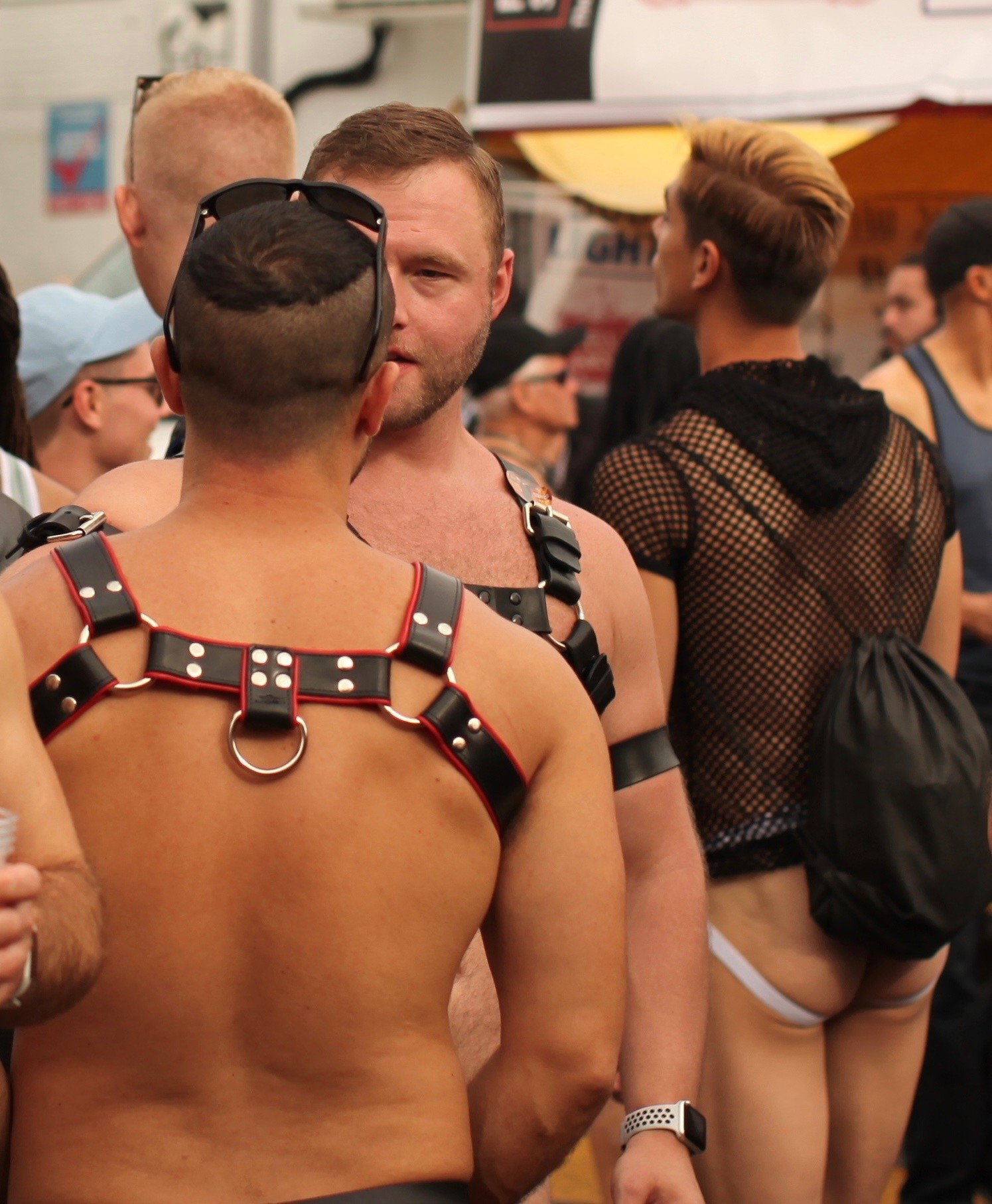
[162,177,389,382]
[523,370,568,387]
[129,75,163,184]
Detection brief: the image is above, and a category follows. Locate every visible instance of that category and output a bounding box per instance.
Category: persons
[19,103,713,1204]
[0,63,946,570]
[588,129,992,1204]
[0,176,622,1204]
[0,587,103,1156]
[859,199,992,1204]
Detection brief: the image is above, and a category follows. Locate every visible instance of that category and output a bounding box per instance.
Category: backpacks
[656,412,992,958]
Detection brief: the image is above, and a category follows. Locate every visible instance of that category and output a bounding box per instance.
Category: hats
[923,197,992,296]
[464,324,587,398]
[15,280,169,424]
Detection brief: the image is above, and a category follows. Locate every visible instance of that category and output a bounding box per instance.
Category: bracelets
[7,924,39,1009]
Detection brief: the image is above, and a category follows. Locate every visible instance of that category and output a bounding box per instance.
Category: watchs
[621,1099,707,1157]
